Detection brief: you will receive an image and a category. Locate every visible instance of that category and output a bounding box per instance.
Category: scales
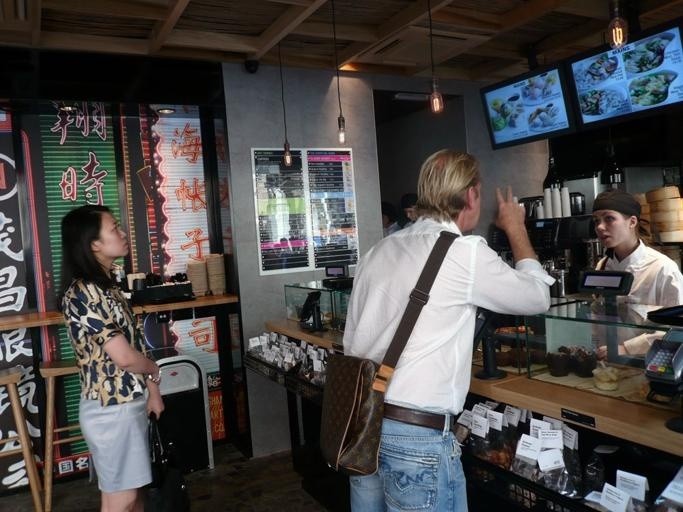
[578,270,648,369]
[325,264,349,332]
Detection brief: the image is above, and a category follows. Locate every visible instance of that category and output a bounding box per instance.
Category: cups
[535,187,571,220]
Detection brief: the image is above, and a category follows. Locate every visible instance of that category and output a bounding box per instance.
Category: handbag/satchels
[319,354,386,476]
[148,413,189,511]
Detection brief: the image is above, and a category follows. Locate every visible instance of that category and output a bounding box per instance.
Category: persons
[381,201,400,238]
[341,149,551,512]
[56,206,165,512]
[589,191,682,362]
[401,193,421,225]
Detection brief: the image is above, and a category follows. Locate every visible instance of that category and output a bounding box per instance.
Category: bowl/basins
[547,352,596,378]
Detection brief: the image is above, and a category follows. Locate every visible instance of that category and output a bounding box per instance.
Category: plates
[572,32,678,117]
[185,254,225,297]
[523,95,545,107]
[529,126,554,132]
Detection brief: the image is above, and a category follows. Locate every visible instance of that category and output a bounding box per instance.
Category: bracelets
[147,370,160,381]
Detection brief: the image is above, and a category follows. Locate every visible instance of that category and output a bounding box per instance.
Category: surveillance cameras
[245,60,260,73]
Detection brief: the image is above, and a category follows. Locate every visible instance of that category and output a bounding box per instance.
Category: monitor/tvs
[564,15,683,133]
[480,61,574,151]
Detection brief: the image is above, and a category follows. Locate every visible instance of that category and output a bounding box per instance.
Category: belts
[384,403,454,432]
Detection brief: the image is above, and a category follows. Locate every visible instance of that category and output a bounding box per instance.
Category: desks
[0,291,252,462]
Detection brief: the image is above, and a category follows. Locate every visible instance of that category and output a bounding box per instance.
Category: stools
[0,359,79,510]
